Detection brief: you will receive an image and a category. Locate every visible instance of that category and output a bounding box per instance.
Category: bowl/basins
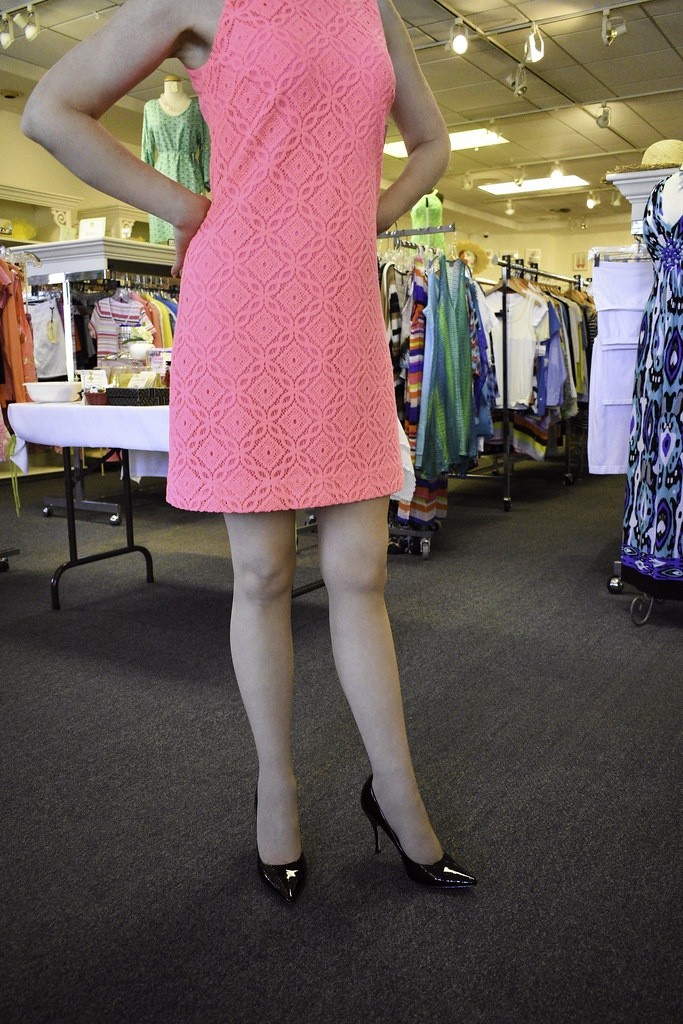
[22,381,82,403]
[84,392,107,405]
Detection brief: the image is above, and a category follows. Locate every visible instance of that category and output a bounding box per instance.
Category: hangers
[376,221,599,310]
[1,246,182,304]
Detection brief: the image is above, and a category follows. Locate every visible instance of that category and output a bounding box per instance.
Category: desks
[7,399,413,610]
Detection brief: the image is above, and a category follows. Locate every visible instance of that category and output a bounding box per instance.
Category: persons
[22,0,477,901]
[141,75,211,244]
[620,163,683,582]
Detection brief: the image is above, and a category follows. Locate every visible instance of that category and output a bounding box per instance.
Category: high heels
[359,773,477,889]
[254,779,307,905]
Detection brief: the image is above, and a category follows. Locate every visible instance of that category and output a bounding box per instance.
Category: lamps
[462,175,474,192]
[14,5,41,41]
[446,19,470,55]
[595,102,611,129]
[0,13,15,48]
[523,21,544,64]
[505,188,624,216]
[507,64,529,98]
[599,11,627,46]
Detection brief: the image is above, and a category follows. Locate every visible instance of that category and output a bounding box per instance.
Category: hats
[607,139,683,174]
